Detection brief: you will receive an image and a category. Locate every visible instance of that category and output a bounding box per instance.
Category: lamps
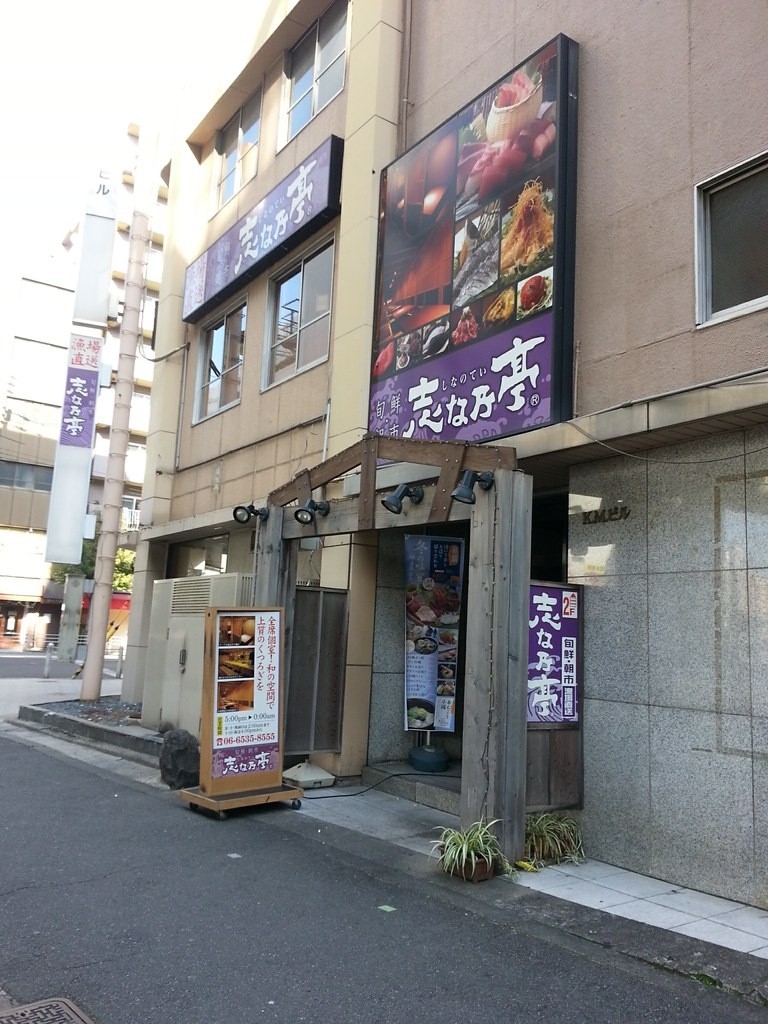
[382,483,424,514]
[233,505,269,524]
[294,499,330,524]
[451,469,494,504]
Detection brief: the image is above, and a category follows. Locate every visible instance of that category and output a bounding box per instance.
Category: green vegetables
[500,193,555,283]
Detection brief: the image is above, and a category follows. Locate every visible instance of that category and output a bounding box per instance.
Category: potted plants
[427,814,521,884]
[524,808,588,871]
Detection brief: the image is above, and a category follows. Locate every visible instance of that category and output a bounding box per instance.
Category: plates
[427,326,448,354]
[517,266,553,319]
[396,356,410,369]
